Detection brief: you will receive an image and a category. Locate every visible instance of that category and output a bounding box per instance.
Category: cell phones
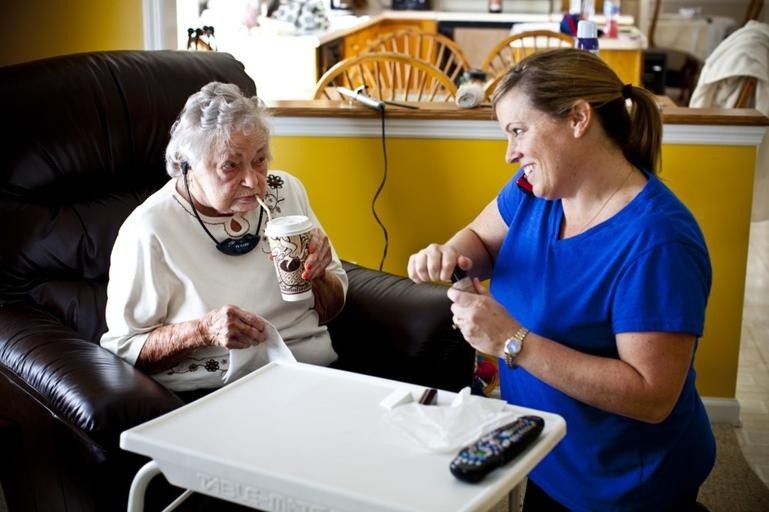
[516,173,532,195]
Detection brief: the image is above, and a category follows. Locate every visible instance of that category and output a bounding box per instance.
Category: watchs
[504,326,530,368]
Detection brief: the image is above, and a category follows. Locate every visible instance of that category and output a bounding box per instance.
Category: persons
[405,50,717,510]
[98,80,349,406]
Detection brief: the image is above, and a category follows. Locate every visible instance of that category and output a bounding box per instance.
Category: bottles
[447,263,477,299]
[574,18,598,58]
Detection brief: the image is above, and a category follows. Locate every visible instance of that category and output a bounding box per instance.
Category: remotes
[450,416,544,485]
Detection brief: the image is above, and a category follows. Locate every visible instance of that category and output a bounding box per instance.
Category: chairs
[343,30,472,103]
[311,50,468,100]
[0,51,476,512]
[485,30,578,102]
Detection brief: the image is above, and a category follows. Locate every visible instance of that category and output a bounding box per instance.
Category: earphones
[181,162,188,175]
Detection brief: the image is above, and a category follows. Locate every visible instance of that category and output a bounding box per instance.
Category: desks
[120,358,567,512]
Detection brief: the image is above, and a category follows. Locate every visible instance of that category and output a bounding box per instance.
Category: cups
[263,216,319,303]
